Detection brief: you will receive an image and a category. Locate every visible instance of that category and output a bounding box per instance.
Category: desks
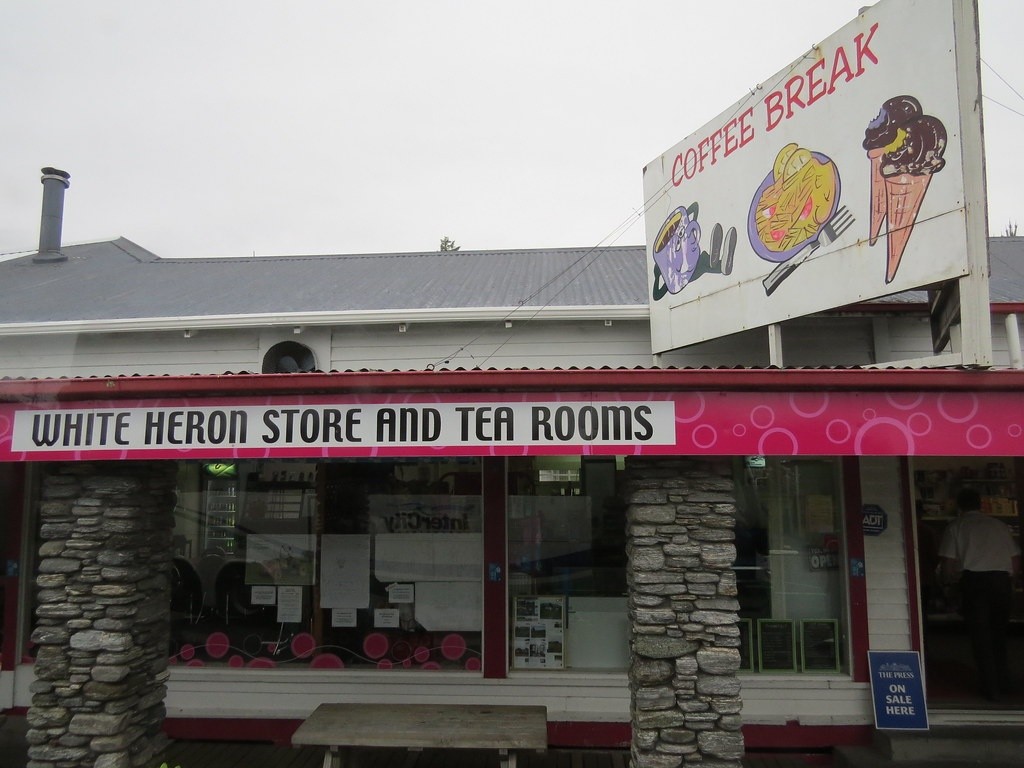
[290,703,548,768]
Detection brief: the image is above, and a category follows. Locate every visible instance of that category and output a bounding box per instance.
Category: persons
[933,489,1024,702]
[915,500,937,587]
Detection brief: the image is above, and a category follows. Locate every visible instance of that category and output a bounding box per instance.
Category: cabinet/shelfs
[920,477,1020,521]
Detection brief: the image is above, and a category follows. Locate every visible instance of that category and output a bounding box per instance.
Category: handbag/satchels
[941,520,961,585]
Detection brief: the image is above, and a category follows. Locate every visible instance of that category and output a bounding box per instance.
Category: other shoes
[976,687,1002,702]
[1000,687,1008,696]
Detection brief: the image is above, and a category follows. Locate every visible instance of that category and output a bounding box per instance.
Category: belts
[963,571,1008,576]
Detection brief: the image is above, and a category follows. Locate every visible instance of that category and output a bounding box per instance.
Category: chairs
[172,556,208,624]
[213,560,277,626]
